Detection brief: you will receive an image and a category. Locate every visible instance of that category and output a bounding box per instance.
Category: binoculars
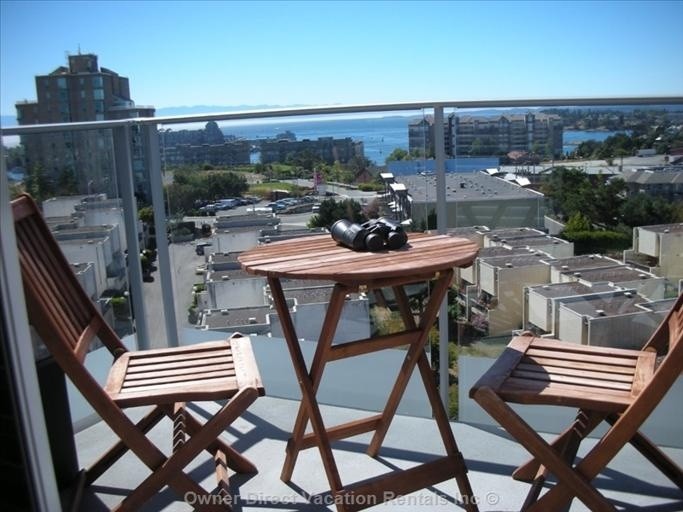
[330,217,408,251]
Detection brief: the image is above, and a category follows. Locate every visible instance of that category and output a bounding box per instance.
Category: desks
[237,233,481,512]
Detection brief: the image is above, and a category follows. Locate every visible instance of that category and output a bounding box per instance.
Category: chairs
[469,289,683,512]
[10,193,266,512]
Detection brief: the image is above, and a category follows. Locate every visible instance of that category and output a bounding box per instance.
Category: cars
[194,192,263,215]
[265,192,324,215]
